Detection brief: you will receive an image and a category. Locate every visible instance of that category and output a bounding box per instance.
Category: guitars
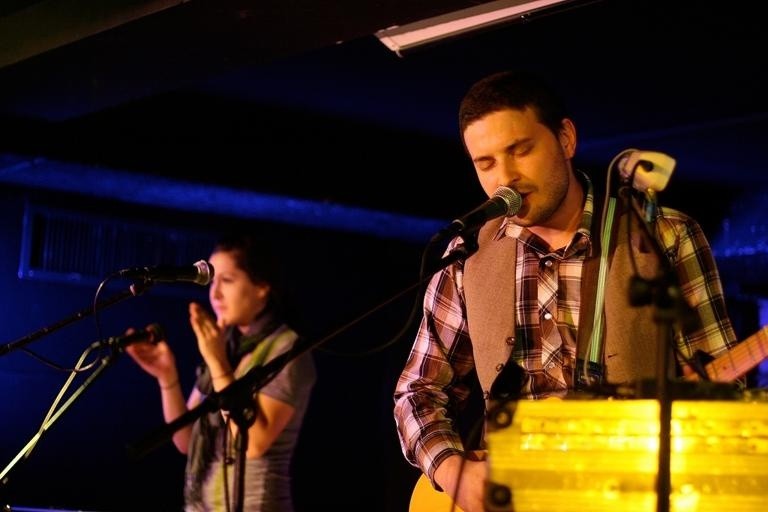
[409,320,767,512]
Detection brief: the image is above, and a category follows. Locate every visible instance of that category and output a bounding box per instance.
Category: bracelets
[160,380,180,390]
[211,369,231,383]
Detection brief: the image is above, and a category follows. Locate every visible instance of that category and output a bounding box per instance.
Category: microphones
[119,259,215,285]
[432,186,523,242]
[107,323,166,344]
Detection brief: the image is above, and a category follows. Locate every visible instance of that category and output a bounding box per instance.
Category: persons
[125,237,318,512]
[391,63,749,512]
